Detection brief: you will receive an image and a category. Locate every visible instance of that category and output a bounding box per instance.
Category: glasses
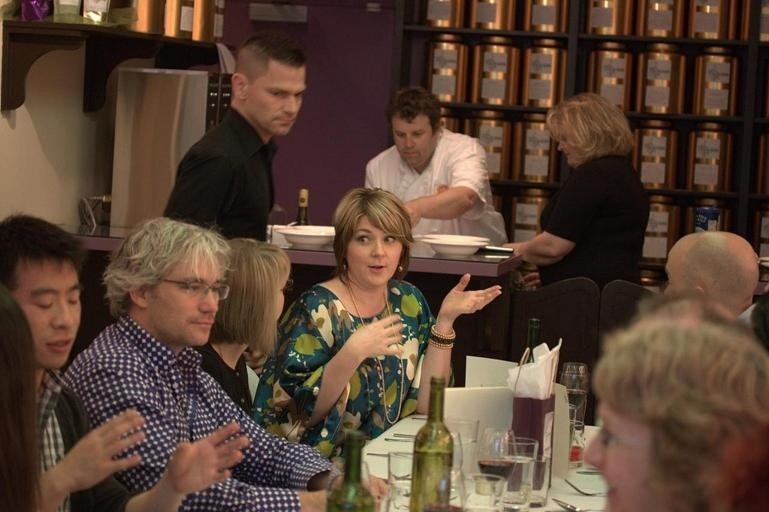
[155,276,232,302]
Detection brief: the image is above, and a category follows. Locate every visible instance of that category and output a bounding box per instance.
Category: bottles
[525,317,542,363]
[294,188,310,226]
[409,372,455,510]
[325,430,377,512]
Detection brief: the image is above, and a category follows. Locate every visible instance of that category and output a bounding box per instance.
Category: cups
[361,449,410,512]
[457,360,590,510]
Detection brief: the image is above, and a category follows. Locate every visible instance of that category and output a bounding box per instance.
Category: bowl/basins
[421,232,491,256]
[277,222,337,249]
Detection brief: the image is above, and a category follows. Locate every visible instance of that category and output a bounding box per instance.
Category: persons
[198,235,293,423]
[164,35,310,244]
[499,88,649,288]
[0,287,51,512]
[0,209,250,511]
[254,185,506,458]
[663,230,760,325]
[751,292,769,342]
[60,215,386,511]
[363,85,507,250]
[581,312,769,511]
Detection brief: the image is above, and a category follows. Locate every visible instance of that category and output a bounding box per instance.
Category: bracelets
[427,322,458,350]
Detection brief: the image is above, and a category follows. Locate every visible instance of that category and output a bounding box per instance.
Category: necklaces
[341,276,414,425]
